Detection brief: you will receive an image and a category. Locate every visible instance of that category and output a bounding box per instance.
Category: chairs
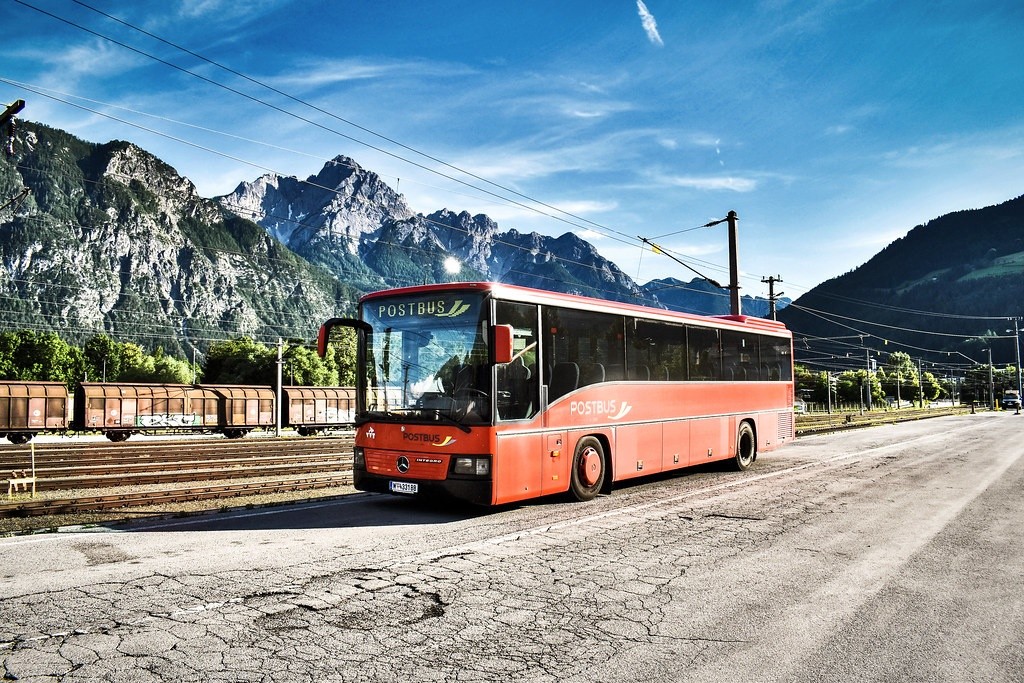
[450,356,780,419]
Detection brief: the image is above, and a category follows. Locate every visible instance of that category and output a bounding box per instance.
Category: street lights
[982,347,994,411]
[1006,317,1023,409]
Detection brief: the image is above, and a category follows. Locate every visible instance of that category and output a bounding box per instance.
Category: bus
[317,281,798,506]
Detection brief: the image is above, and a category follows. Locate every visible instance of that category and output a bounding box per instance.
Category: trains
[1,381,408,445]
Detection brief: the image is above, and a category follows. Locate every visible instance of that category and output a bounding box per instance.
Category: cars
[1002,390,1022,411]
